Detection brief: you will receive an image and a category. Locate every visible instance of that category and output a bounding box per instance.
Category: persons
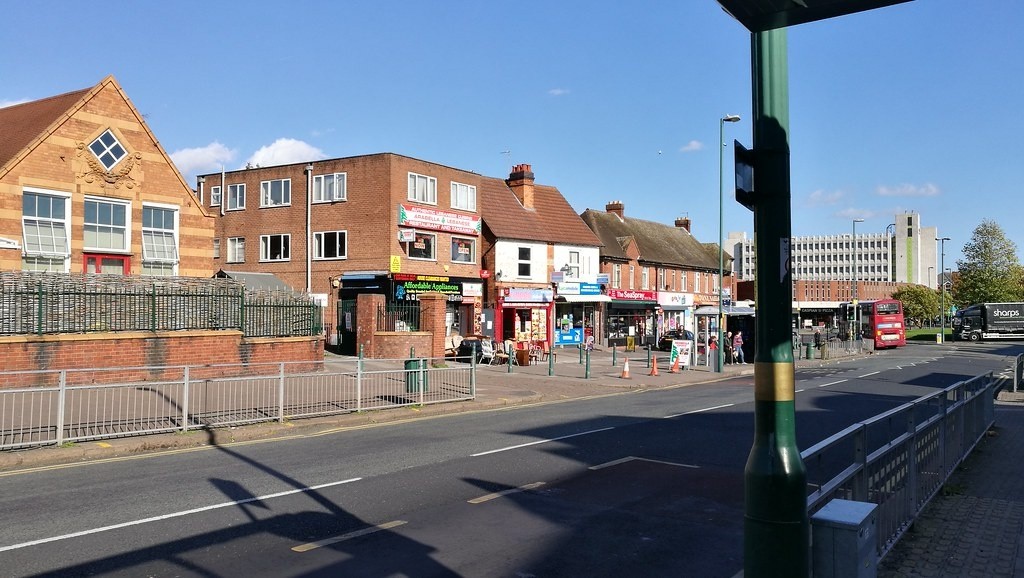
[676,325,687,340]
[814,329,821,351]
[708,331,747,365]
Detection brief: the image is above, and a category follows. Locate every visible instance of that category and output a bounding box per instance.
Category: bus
[836,299,906,349]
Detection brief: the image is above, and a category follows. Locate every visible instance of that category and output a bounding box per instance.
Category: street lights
[852,218,864,342]
[935,237,951,344]
[718,114,740,373]
[927,266,934,329]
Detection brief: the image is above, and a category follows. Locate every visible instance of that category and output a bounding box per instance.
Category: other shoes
[742,362,747,365]
[736,362,740,364]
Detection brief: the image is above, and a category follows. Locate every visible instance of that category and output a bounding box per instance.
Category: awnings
[558,294,612,304]
[611,299,661,310]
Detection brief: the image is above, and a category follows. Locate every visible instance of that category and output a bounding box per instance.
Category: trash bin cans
[404,359,427,393]
[806,342,815,360]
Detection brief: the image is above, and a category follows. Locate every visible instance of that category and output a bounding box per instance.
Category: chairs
[452,334,557,366]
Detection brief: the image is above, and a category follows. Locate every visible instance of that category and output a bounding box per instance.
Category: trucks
[951,302,1024,343]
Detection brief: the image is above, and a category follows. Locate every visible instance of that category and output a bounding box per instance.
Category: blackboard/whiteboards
[804,319,813,327]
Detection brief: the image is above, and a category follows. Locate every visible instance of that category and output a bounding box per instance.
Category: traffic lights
[847,304,856,322]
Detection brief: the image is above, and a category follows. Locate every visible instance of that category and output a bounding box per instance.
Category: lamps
[566,268,573,275]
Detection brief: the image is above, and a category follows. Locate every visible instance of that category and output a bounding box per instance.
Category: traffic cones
[649,354,661,376]
[670,354,681,374]
[617,356,631,380]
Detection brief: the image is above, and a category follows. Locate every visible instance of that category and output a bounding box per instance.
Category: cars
[903,313,947,328]
[658,328,703,353]
